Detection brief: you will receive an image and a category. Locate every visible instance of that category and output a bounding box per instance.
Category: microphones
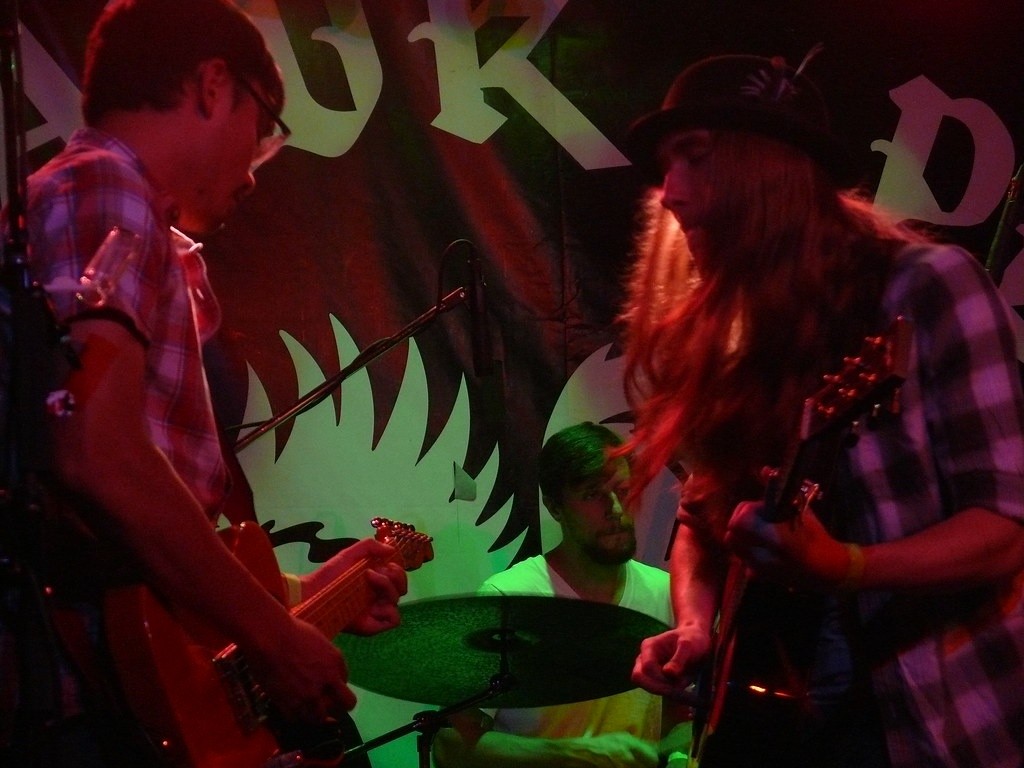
[468,242,495,379]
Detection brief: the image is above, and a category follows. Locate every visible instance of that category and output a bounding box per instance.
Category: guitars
[106,512,439,768]
[688,311,916,768]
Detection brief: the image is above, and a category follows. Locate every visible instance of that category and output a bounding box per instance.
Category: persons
[28,0,435,767]
[597,52,1024,767]
[431,421,693,768]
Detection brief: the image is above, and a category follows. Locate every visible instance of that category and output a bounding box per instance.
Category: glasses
[232,67,293,174]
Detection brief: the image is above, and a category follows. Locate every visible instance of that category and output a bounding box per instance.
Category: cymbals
[326,589,681,713]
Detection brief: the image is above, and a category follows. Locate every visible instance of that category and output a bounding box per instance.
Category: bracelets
[841,541,864,592]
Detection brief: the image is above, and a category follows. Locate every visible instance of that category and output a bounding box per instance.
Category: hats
[625,52,847,169]
